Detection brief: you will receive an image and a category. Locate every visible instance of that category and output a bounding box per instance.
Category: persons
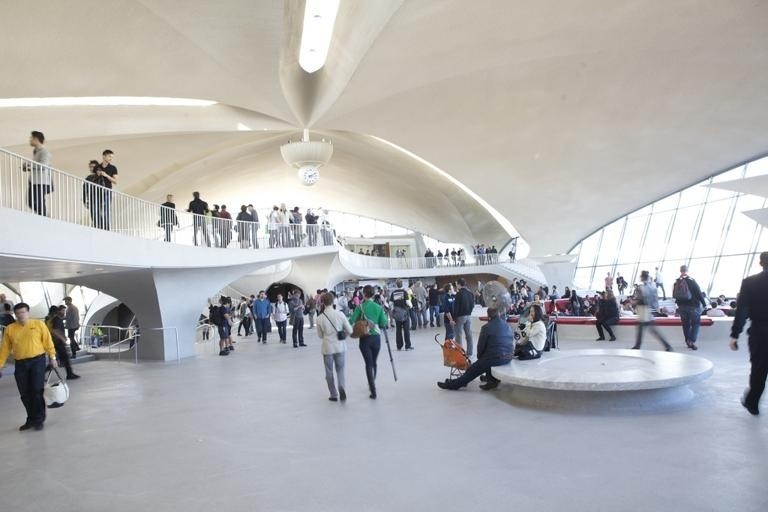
[19,131,50,216]
[726,250,768,421]
[184,192,336,248]
[337,235,498,268]
[1,294,82,379]
[0,301,60,430]
[474,264,707,391]
[198,278,475,403]
[508,243,515,263]
[161,193,180,242]
[129,323,140,349]
[90,323,104,348]
[83,150,118,231]
[701,292,739,317]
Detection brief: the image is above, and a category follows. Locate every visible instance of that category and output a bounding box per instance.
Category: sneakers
[329,398,337,401]
[293,344,297,348]
[219,346,234,355]
[437,382,457,390]
[66,374,80,380]
[19,422,43,431]
[685,337,697,350]
[299,344,306,346]
[339,389,346,400]
[479,383,497,389]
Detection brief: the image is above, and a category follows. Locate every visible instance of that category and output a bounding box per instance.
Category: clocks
[297,165,320,187]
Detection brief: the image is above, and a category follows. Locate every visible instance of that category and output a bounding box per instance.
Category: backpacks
[674,276,692,302]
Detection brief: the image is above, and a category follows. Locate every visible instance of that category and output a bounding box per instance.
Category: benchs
[479,298,714,340]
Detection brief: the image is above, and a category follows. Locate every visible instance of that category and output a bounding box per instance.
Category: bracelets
[49,357,57,360]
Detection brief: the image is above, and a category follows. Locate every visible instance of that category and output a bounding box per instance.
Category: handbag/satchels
[514,342,541,360]
[350,321,368,338]
[391,306,408,323]
[43,368,70,408]
[338,331,345,340]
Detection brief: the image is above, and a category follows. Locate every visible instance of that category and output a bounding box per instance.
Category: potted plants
[91,328,108,346]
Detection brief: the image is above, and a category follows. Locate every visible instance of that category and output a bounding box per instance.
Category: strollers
[543,311,559,352]
[434,333,486,383]
